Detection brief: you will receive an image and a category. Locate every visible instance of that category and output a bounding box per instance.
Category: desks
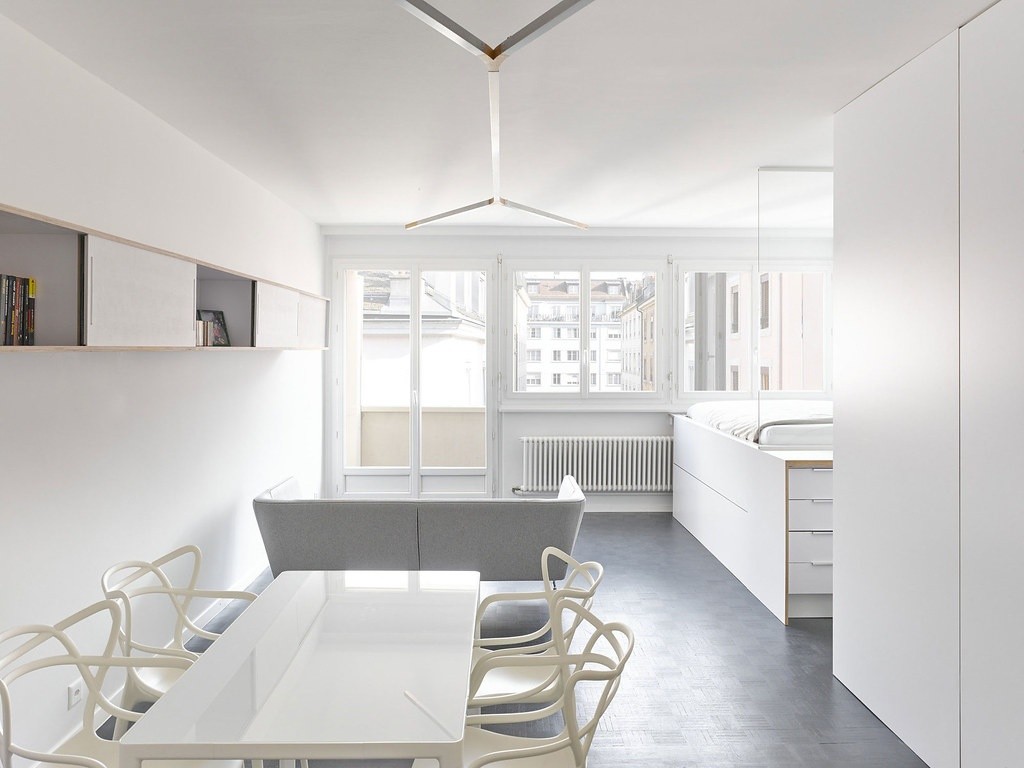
[119,570,480,768]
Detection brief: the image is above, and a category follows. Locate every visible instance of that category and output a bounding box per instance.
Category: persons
[213,321,226,345]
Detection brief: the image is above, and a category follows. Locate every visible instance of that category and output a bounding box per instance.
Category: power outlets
[68,676,84,710]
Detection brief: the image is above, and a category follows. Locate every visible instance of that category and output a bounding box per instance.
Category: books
[0,274,38,347]
[196,320,215,346]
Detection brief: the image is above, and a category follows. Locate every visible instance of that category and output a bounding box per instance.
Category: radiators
[511,437,673,496]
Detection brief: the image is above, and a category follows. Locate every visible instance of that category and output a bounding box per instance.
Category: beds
[673,400,834,625]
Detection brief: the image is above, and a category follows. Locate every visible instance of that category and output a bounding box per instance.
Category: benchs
[252,475,586,591]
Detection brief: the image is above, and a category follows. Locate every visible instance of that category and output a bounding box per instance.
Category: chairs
[102,544,309,768]
[0,599,246,768]
[413,597,634,768]
[467,545,605,730]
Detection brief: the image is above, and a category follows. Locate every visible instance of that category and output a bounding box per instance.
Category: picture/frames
[198,309,231,347]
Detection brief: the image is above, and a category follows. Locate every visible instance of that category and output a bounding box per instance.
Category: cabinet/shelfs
[0,204,329,350]
